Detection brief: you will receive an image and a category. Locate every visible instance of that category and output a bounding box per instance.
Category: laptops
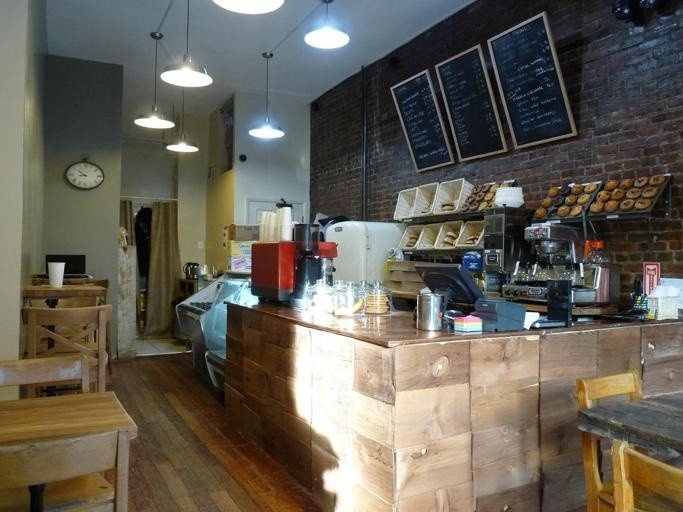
[45,255,86,277]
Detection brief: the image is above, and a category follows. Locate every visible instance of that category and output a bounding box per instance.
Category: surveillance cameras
[238,153,248,163]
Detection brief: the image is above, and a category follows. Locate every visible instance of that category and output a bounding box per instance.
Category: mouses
[86,275,94,278]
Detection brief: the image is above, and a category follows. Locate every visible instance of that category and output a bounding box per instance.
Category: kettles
[182,262,197,280]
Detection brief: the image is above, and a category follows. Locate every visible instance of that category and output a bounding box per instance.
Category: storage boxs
[455,221,487,249]
[228,224,260,240]
[219,240,257,274]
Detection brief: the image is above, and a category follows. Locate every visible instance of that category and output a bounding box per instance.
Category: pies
[404,201,481,247]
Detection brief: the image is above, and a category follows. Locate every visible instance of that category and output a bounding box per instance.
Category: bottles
[197,264,222,281]
[308,279,391,317]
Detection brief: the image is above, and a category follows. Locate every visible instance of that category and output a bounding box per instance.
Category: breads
[534,175,666,218]
[461,182,510,212]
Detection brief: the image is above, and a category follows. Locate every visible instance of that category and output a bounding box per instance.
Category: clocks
[65,154,104,190]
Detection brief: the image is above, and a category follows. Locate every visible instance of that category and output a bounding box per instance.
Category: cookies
[351,294,390,316]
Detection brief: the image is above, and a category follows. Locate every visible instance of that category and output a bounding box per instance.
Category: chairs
[1,256,139,511]
[576,368,648,512]
[610,437,683,512]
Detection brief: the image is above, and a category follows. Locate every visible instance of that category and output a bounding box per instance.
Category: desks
[577,392,683,453]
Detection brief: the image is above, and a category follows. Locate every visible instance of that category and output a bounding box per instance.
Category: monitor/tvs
[413,262,485,329]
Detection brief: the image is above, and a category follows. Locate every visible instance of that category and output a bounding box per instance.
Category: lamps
[165,88,199,153]
[304,0,350,50]
[248,52,285,139]
[160,0,214,88]
[134,32,176,129]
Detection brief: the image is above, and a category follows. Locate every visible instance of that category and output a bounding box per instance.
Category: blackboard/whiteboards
[390,12,578,173]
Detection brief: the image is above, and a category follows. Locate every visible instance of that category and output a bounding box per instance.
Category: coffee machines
[501,221,622,307]
[483,207,534,299]
[289,225,324,310]
[251,242,343,305]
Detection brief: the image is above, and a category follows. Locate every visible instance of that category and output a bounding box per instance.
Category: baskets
[454,220,484,250]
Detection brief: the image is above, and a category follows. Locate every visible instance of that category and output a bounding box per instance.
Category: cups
[416,293,443,333]
[47,262,65,290]
[259,207,295,243]
